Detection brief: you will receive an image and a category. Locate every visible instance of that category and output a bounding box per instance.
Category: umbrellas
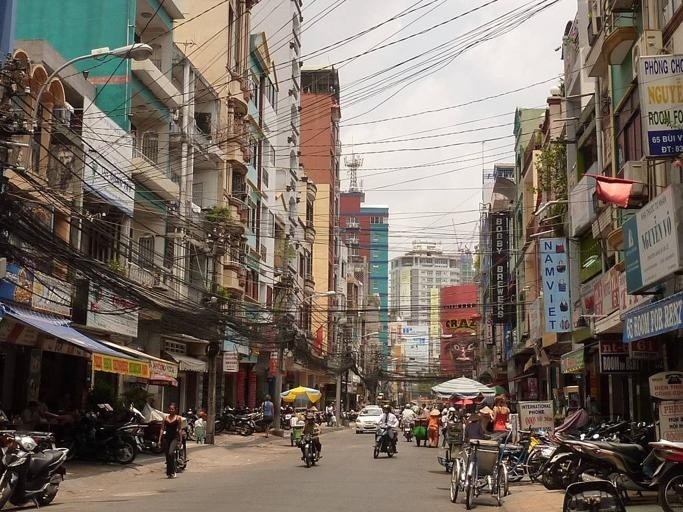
[280,386,322,408]
[430,374,496,414]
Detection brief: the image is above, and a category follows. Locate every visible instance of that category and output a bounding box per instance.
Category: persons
[157,404,183,478]
[195,411,207,445]
[401,394,511,449]
[325,400,335,426]
[298,413,322,462]
[376,405,399,455]
[261,393,276,437]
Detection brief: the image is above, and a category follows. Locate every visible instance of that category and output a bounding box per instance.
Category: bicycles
[448,437,509,511]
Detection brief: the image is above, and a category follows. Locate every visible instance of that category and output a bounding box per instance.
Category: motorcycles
[498,408,682,511]
[298,428,321,469]
[443,416,463,451]
[0,431,70,510]
[402,418,414,443]
[293,407,353,425]
[0,399,294,473]
[373,430,398,460]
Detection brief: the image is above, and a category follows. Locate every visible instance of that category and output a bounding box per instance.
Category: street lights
[0,43,155,279]
[375,356,400,402]
[439,333,497,385]
[335,331,379,429]
[273,290,336,432]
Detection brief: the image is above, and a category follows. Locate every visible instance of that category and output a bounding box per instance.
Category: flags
[597,175,631,207]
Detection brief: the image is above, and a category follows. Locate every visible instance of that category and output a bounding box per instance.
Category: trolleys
[414,414,439,447]
[289,418,305,446]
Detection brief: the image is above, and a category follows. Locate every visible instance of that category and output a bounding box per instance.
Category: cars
[355,403,385,435]
